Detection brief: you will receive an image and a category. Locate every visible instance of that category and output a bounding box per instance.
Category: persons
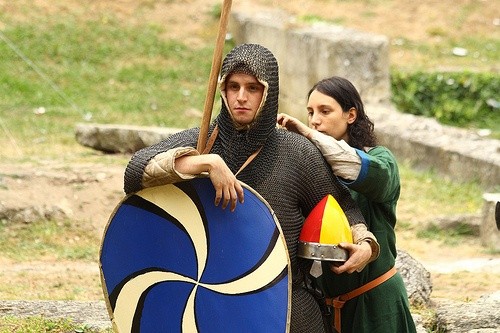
[275,76,418,333]
[123,44,382,333]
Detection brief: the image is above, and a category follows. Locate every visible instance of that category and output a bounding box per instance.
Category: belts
[326,264,398,333]
